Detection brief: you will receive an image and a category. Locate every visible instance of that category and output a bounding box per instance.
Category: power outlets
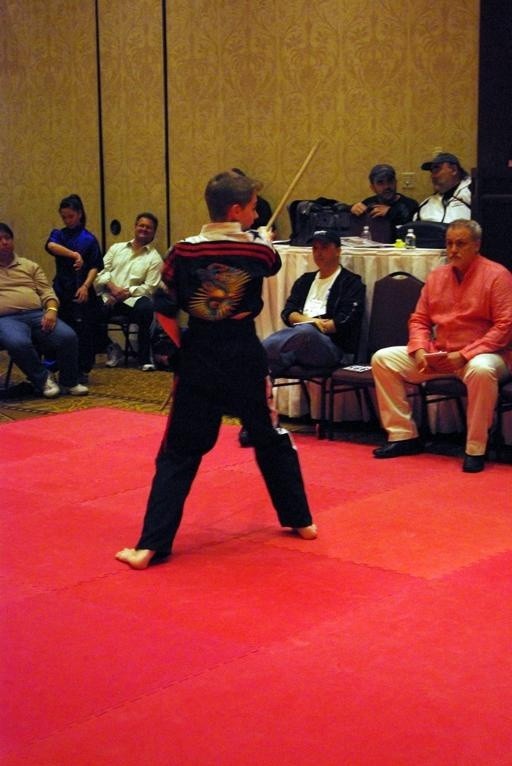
[400,172,415,188]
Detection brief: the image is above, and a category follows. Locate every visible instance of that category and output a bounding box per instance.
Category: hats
[421,152,462,171]
[305,227,342,246]
[371,164,397,180]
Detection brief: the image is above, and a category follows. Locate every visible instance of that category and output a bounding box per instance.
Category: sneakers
[42,369,61,399]
[105,343,127,367]
[60,383,90,397]
[136,360,157,371]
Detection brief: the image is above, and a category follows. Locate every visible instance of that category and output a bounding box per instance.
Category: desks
[257,244,463,434]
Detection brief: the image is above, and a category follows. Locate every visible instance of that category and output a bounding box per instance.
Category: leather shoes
[462,454,485,472]
[374,441,426,458]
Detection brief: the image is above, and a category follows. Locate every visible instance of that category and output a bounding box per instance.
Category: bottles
[362,226,371,240]
[405,229,416,248]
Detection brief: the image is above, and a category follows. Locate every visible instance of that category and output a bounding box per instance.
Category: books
[340,235,396,247]
[342,365,371,372]
[292,320,322,331]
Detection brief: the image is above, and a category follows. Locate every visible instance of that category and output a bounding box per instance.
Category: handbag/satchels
[398,219,449,250]
[290,197,362,247]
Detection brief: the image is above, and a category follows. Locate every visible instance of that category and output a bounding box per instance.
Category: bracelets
[46,305,58,313]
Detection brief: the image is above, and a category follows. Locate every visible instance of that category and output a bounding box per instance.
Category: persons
[0,222,89,396]
[94,212,164,371]
[411,153,473,225]
[229,168,276,233]
[372,220,512,472]
[114,170,318,570]
[347,164,421,225]
[42,194,104,384]
[239,230,364,448]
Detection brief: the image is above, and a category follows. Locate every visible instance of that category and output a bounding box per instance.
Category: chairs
[422,378,512,462]
[270,273,366,438]
[0,341,47,397]
[107,310,152,364]
[329,271,426,439]
